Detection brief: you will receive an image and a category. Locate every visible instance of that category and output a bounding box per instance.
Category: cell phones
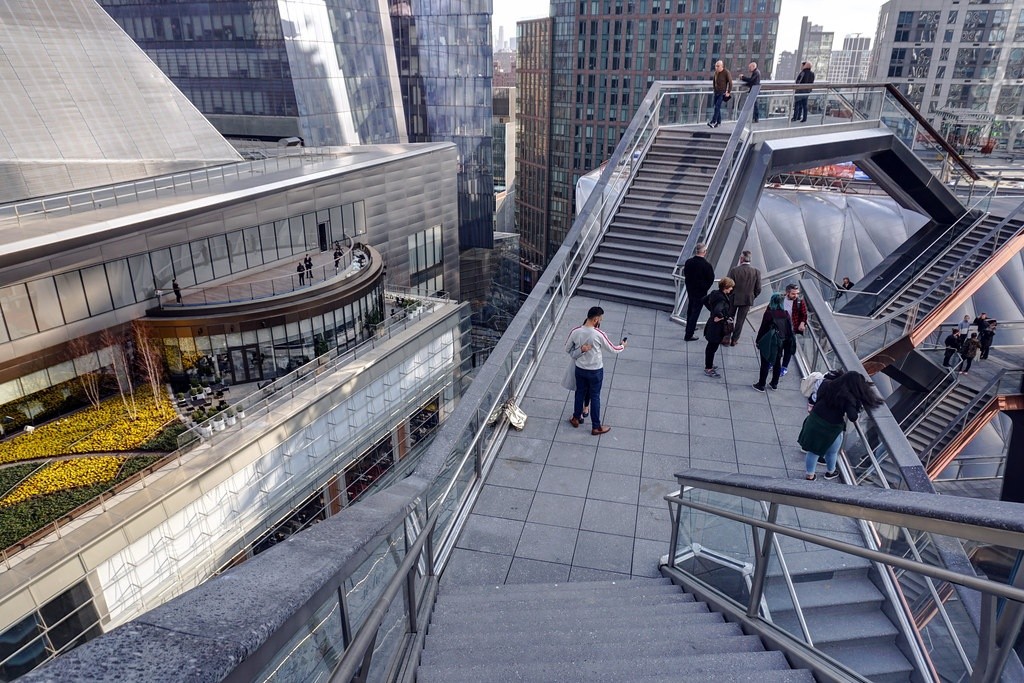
[622,337,627,342]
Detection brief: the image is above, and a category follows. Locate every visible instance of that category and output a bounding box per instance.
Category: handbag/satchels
[723,321,734,335]
[487,402,505,426]
[796,300,804,336]
[505,396,528,432]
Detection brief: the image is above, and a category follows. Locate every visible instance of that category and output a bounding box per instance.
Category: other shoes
[583,412,588,418]
[714,123,722,127]
[707,122,715,128]
[721,336,730,346]
[695,328,698,330]
[592,425,610,435]
[570,417,579,427]
[684,337,699,341]
[731,341,737,346]
[580,419,584,423]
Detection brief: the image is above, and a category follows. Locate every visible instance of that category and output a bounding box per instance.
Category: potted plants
[191,404,245,437]
[189,386,205,400]
[176,392,186,403]
[403,298,423,320]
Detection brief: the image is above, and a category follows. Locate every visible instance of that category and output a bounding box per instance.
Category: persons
[389,309,396,318]
[684,243,715,342]
[840,278,855,298]
[790,62,815,123]
[751,284,808,392]
[251,234,256,250]
[333,239,344,268]
[227,240,234,263]
[396,296,400,303]
[739,62,762,124]
[172,278,181,303]
[721,250,762,347]
[153,275,163,289]
[942,154,953,183]
[796,369,886,481]
[296,254,313,285]
[959,145,965,155]
[702,276,735,378]
[947,131,977,145]
[707,59,733,129]
[564,306,628,435]
[943,312,998,373]
[200,244,209,265]
[401,298,404,303]
[988,139,998,148]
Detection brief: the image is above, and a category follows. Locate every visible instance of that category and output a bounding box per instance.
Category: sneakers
[817,458,827,465]
[769,364,773,372]
[769,382,777,390]
[705,366,721,379]
[806,474,817,481]
[800,447,809,454]
[824,471,839,479]
[779,368,788,377]
[752,383,765,392]
